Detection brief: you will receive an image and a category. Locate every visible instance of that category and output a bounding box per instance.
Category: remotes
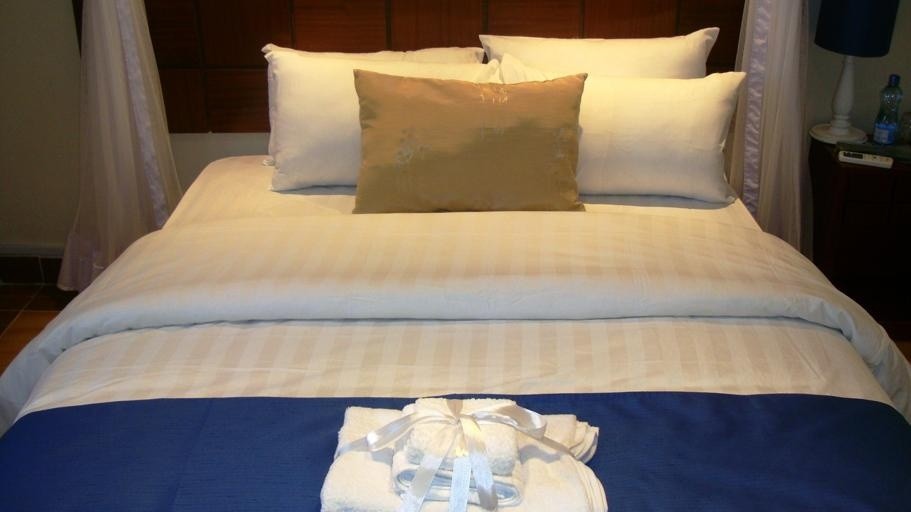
[838,148,895,173]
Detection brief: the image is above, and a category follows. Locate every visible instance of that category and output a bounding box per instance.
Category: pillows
[261,43,485,168]
[266,49,505,193]
[350,67,589,215]
[501,54,749,206]
[475,27,721,82]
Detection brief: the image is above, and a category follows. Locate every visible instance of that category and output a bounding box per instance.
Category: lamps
[810,0,897,146]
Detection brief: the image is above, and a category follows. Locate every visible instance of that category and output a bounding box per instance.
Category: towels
[316,392,608,512]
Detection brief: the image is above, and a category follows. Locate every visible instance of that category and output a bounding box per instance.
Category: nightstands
[806,136,911,341]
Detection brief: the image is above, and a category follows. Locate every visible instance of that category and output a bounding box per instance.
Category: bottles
[872,74,903,145]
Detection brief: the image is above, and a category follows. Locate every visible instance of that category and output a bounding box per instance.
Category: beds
[1,154,910,512]
